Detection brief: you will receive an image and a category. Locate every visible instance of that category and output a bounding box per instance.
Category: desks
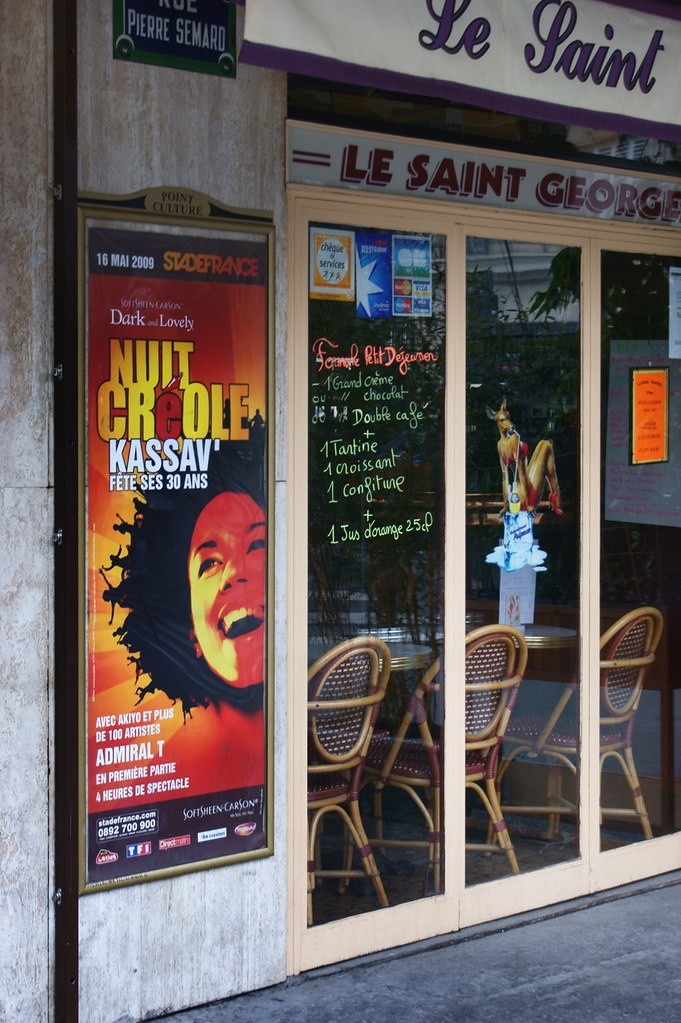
[309,643,433,672]
[466,625,576,651]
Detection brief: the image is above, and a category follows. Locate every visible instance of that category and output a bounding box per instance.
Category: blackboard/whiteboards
[307,326,446,545]
[602,336,681,530]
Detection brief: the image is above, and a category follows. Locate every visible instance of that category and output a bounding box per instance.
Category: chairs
[486,606,664,858]
[307,637,391,928]
[339,625,529,896]
